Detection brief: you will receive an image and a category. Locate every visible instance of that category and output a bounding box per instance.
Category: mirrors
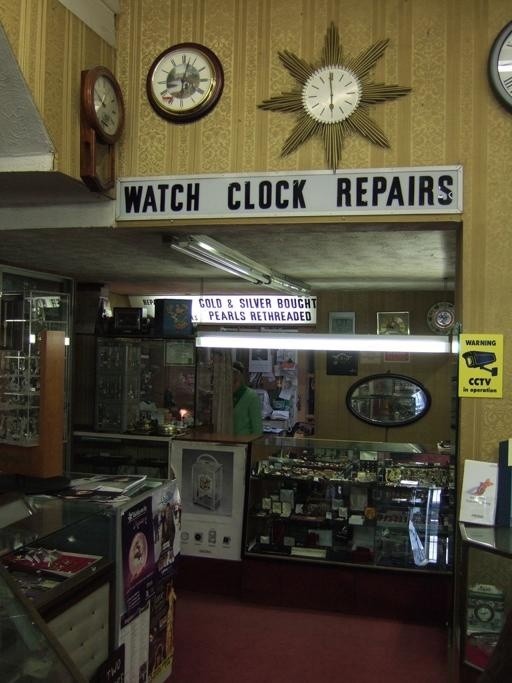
[345,370,432,427]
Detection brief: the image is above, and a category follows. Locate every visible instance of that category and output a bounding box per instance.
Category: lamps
[165,230,312,295]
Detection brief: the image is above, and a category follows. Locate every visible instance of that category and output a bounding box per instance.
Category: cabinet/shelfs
[95,341,143,433]
[3,353,42,446]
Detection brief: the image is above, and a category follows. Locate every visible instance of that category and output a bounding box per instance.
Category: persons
[232,361,262,435]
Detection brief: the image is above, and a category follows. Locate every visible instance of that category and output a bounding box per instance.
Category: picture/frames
[161,340,196,368]
[326,351,358,377]
[113,306,143,331]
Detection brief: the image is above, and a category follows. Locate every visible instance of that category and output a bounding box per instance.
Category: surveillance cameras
[462,351,498,368]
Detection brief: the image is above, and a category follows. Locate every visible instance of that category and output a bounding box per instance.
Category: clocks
[375,309,412,336]
[266,22,415,170]
[146,40,225,124]
[80,64,125,192]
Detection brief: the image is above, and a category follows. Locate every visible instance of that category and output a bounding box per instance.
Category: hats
[232,362,246,375]
